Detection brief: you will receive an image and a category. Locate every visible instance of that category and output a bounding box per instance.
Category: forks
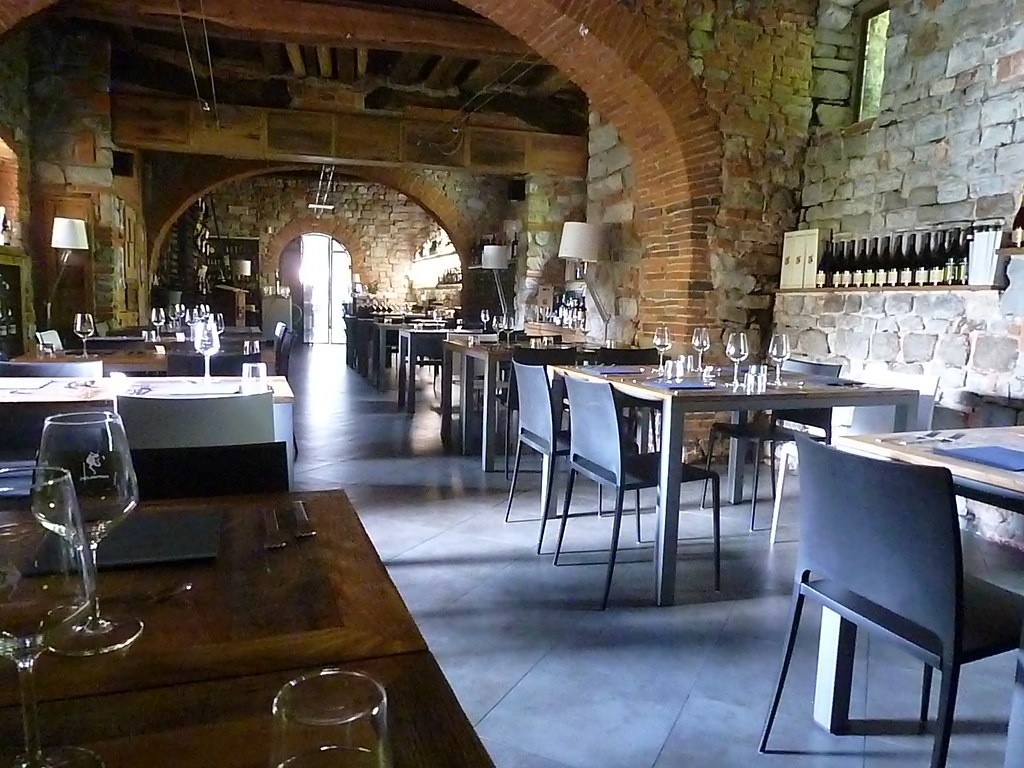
[896,432,966,446]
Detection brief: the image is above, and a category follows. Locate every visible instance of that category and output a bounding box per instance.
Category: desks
[441,337,610,475]
[539,364,920,609]
[0,487,499,768]
[813,426,1024,733]
[0,322,295,491]
[397,328,483,413]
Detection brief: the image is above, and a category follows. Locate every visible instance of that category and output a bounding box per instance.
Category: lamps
[557,222,612,329]
[232,259,253,289]
[46,217,90,329]
[482,243,512,330]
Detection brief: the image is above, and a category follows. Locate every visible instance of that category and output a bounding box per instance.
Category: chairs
[340,302,843,610]
[261,321,287,376]
[275,329,299,457]
[758,432,1024,768]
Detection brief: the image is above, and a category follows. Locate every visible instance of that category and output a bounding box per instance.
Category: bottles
[554,294,586,331]
[816,225,1001,287]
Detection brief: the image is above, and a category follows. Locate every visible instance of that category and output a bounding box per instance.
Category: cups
[243,340,260,356]
[241,362,268,395]
[267,667,392,768]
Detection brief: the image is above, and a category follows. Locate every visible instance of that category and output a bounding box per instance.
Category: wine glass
[169,303,225,342]
[151,308,165,343]
[652,327,673,374]
[767,333,791,387]
[691,327,710,373]
[0,462,106,768]
[194,322,221,386]
[725,332,748,387]
[492,316,505,346]
[480,309,490,331]
[74,313,98,359]
[875,430,942,443]
[30,410,145,657]
[502,317,515,348]
